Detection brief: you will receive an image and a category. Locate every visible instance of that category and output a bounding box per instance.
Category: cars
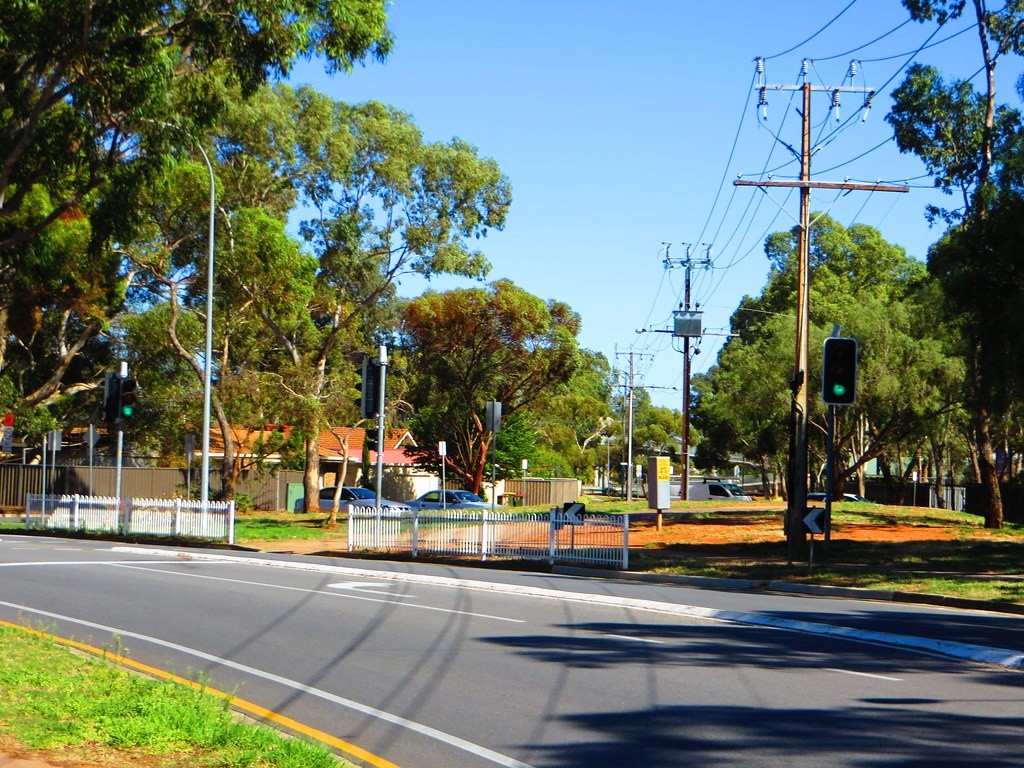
[404,489,504,512]
[293,485,412,514]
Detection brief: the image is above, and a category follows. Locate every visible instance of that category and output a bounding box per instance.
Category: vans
[688,481,753,502]
[806,492,864,503]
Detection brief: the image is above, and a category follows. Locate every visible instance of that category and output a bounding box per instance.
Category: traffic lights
[117,375,137,421]
[822,336,859,407]
[355,357,377,419]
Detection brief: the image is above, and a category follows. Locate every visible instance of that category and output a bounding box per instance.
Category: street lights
[138,117,214,502]
